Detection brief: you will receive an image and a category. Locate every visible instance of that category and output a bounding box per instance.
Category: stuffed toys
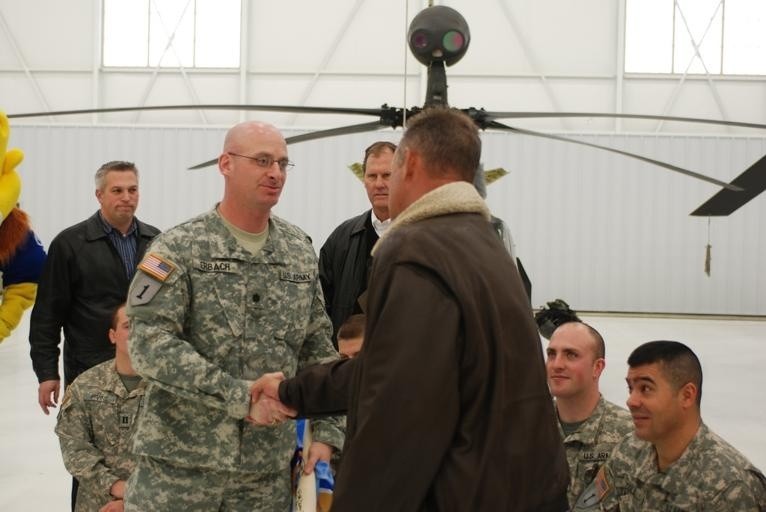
[0,111,47,349]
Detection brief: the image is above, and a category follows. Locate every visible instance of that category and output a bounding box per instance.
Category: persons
[26,159,163,416]
[120,118,347,511]
[53,298,148,511]
[545,319,636,511]
[317,140,400,352]
[249,105,573,512]
[337,312,365,358]
[571,338,766,511]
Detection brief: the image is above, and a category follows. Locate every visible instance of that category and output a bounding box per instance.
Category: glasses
[228,153,295,172]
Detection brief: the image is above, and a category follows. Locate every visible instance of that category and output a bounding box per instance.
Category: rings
[269,418,275,425]
